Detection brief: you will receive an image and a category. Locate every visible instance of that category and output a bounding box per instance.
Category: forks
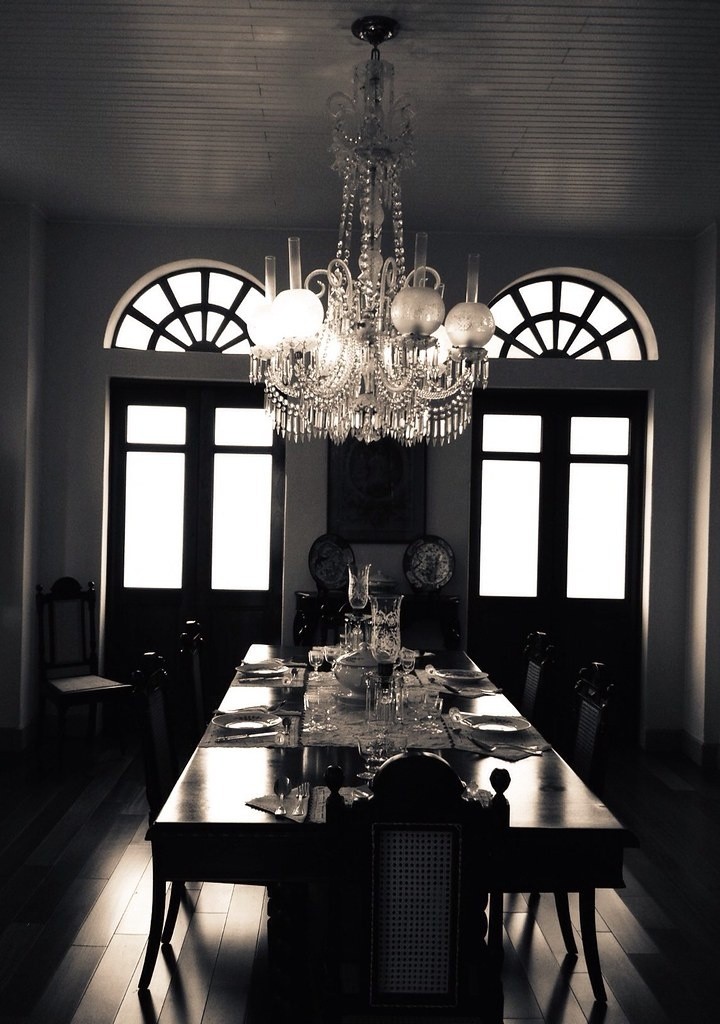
[292,780,309,816]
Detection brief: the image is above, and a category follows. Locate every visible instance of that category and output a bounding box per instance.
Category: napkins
[454,742,552,759]
[211,705,302,716]
[414,669,504,692]
[245,782,310,824]
[231,665,306,687]
[239,657,306,667]
[310,784,375,824]
[441,713,552,745]
[423,687,500,698]
[198,716,301,748]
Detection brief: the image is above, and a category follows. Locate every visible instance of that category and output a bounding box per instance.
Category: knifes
[214,731,279,743]
[236,676,286,682]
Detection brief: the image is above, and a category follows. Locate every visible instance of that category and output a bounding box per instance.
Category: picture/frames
[327,430,428,544]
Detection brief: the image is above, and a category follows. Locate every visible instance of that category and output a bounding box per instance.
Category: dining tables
[137,643,624,1003]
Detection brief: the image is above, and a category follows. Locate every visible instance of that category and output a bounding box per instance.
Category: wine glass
[308,651,324,678]
[350,732,380,780]
[400,650,415,686]
[324,646,341,680]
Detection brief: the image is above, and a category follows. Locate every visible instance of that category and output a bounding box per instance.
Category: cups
[346,561,372,610]
[368,594,405,664]
[364,675,444,762]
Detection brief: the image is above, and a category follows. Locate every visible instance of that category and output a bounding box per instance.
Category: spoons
[273,776,292,816]
[465,734,540,756]
[441,683,503,696]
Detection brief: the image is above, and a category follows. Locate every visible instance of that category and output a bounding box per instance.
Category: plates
[308,533,356,590]
[211,711,282,733]
[403,535,456,592]
[435,668,489,683]
[459,715,532,735]
[235,663,289,678]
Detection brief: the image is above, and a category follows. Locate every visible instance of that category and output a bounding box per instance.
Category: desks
[292,590,461,651]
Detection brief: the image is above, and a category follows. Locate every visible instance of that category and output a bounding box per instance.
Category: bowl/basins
[333,664,395,699]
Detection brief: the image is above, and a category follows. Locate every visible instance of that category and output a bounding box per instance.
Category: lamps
[245,14,496,448]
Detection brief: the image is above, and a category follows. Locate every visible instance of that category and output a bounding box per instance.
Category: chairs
[171,620,233,747]
[504,632,560,729]
[129,647,198,944]
[528,661,619,958]
[35,577,134,777]
[323,750,509,1024]
